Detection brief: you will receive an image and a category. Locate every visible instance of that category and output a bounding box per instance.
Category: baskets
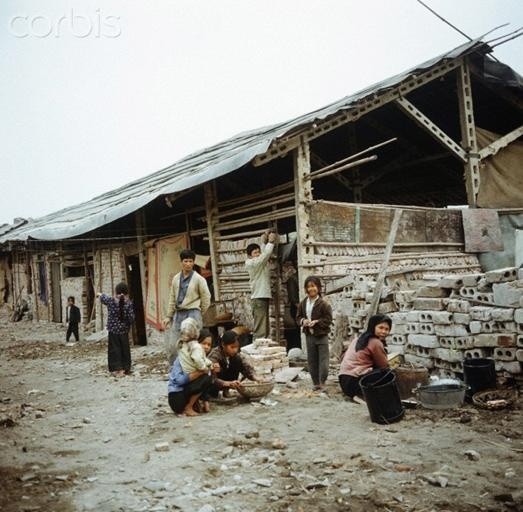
[236,381,274,397]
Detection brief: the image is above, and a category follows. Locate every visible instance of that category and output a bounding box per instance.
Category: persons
[296,276,332,390]
[244,230,276,338]
[339,314,392,403]
[66,297,81,342]
[96,282,135,377]
[161,250,211,366]
[168,317,262,416]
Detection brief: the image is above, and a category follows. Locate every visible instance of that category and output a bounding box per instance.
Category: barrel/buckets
[464,358,497,403]
[359,365,404,425]
[393,367,429,400]
